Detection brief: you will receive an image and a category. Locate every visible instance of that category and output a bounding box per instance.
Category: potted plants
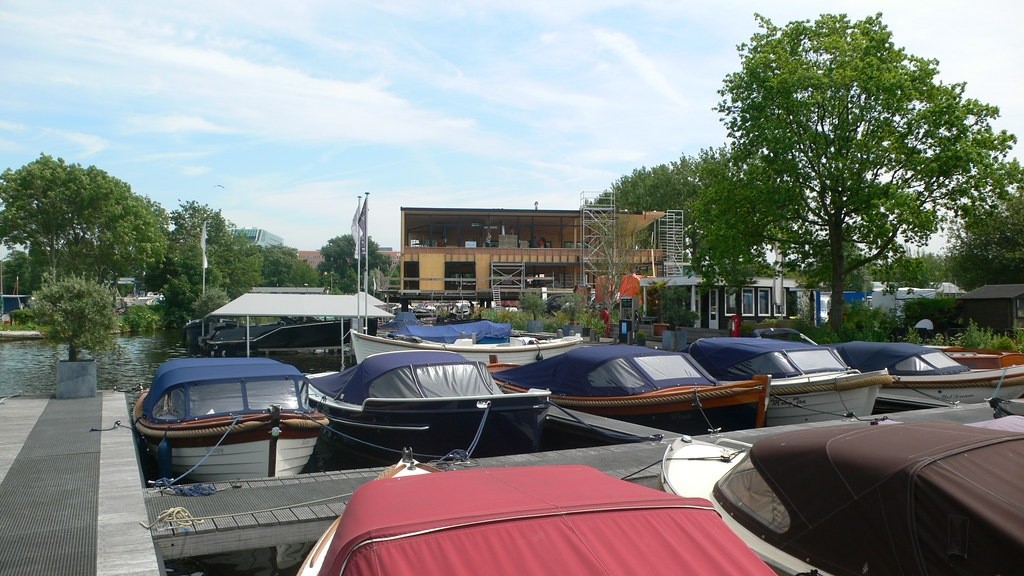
[581,310,594,336]
[657,287,700,350]
[29,270,122,399]
[561,292,583,335]
[590,317,604,341]
[637,332,645,346]
[647,281,669,335]
[519,291,543,332]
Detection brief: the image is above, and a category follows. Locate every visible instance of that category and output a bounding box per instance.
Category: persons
[530,235,547,248]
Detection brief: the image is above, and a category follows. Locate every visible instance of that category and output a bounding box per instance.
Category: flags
[200,222,208,268]
[351,206,360,259]
[358,197,368,256]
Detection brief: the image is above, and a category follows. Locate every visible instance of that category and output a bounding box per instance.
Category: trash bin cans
[619,319,632,344]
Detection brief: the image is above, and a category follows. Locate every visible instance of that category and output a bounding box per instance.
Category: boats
[300,446,777,576]
[660,421,1024,576]
[674,335,896,427]
[484,344,772,438]
[198,291,396,412]
[347,320,584,366]
[755,327,1024,413]
[287,349,553,471]
[132,359,328,486]
